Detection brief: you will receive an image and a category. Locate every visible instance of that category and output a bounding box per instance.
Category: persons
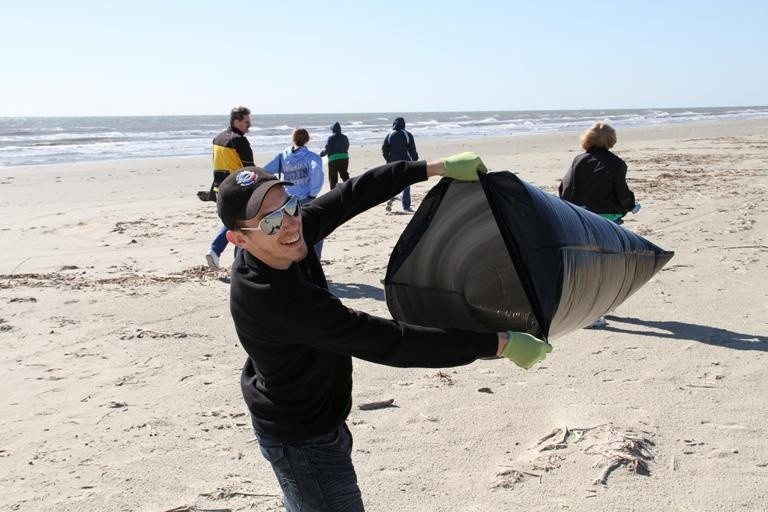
[263,127,324,264]
[558,121,638,329]
[318,120,351,192]
[205,105,257,268]
[214,148,555,511]
[378,117,420,214]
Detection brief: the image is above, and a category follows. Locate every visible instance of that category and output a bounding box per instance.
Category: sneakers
[205,248,221,270]
[385,200,415,212]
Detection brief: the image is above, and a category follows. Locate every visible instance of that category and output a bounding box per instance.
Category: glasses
[237,194,302,235]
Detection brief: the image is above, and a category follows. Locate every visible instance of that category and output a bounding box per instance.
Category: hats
[215,165,295,230]
